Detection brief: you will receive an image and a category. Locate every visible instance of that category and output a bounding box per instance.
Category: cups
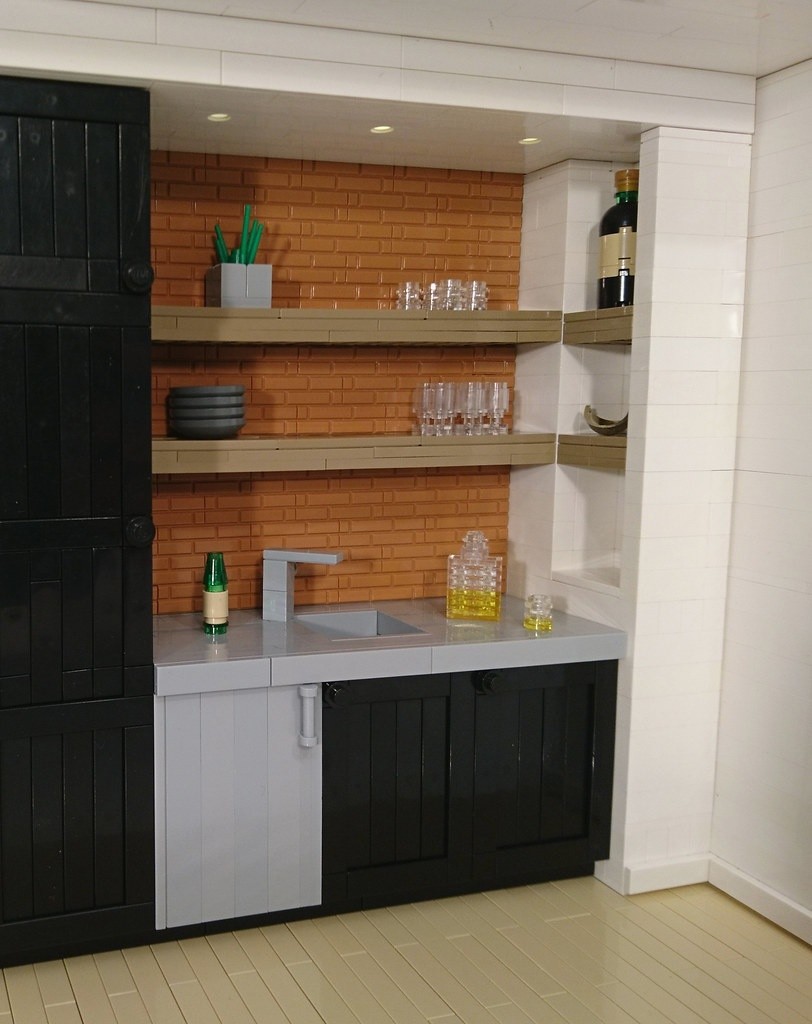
[394,278,489,311]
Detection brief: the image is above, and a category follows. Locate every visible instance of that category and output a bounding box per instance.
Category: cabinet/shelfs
[151,305,565,473]
[555,304,633,476]
[155,683,324,933]
[320,670,480,907]
[470,660,620,884]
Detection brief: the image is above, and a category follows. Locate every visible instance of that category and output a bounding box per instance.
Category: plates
[167,384,246,438]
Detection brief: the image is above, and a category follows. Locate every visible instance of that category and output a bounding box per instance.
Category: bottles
[445,531,503,621]
[596,168,639,308]
[523,595,553,631]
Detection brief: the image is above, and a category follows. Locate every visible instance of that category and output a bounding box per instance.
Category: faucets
[263,545,343,623]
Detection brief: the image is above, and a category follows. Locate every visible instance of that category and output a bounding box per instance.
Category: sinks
[294,609,433,642]
